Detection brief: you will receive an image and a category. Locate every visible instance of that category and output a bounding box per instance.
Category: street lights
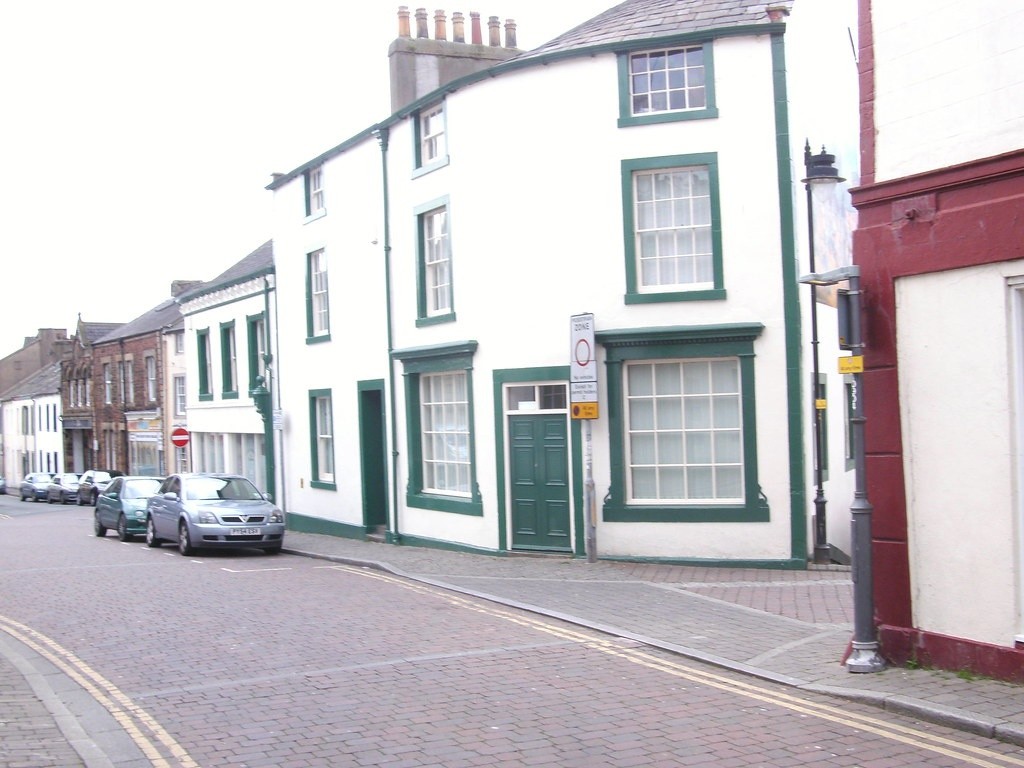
[801,265,887,673]
[800,148,846,565]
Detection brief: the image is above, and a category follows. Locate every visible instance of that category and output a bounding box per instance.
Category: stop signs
[171,427,190,448]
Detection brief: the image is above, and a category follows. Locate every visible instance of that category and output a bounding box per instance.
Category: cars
[47,472,83,504]
[0,476,6,494]
[76,469,128,506]
[93,476,167,541]
[145,472,285,557]
[19,473,54,502]
